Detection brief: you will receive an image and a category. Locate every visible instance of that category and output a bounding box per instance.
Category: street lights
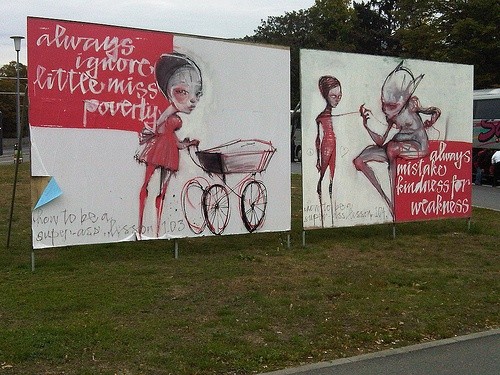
[10,34,25,149]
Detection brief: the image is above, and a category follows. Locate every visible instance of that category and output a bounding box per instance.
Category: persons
[472,148,500,187]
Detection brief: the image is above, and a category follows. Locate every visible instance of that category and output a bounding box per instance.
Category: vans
[469,88,500,186]
